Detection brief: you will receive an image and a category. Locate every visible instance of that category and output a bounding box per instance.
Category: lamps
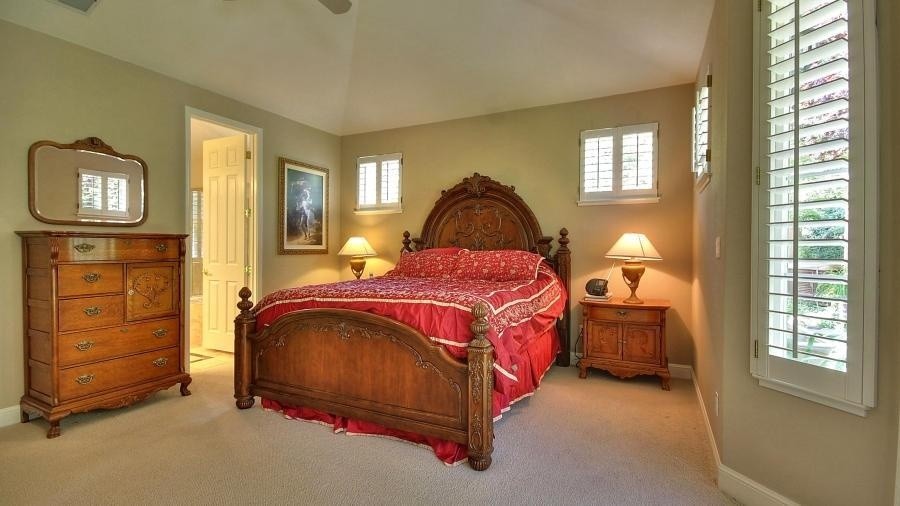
[337,237,378,280]
[604,233,663,304]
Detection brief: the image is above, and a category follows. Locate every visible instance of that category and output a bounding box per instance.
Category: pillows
[387,247,546,283]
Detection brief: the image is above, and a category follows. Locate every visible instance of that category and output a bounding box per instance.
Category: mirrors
[28,137,148,227]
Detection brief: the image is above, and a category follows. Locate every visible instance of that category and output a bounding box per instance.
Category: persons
[297,188,313,239]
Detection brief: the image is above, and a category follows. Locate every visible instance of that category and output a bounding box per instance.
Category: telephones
[586,279,608,296]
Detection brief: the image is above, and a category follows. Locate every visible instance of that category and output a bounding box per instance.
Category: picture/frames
[277,156,329,256]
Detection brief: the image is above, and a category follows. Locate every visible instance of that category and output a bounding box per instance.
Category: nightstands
[578,295,672,391]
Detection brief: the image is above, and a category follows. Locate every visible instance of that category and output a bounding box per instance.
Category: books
[585,296,614,301]
[586,293,613,297]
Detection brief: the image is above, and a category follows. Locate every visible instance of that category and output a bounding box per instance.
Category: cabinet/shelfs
[14,231,192,440]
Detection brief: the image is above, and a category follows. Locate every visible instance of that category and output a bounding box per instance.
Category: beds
[233,172,570,471]
